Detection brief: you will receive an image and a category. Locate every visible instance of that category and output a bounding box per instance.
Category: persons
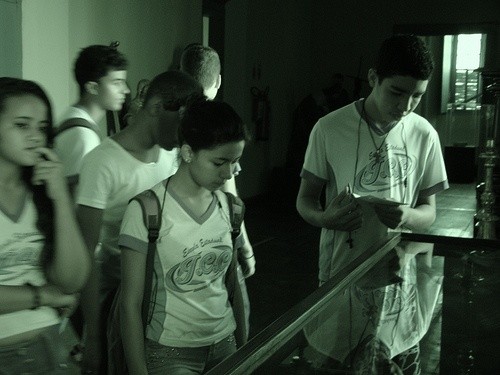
[74,70,211,375]
[295,33,451,290]
[115,100,249,375]
[50,43,132,206]
[301,240,450,375]
[177,44,258,282]
[0,76,91,375]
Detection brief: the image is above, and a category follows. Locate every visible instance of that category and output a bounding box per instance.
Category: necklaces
[358,96,396,161]
[354,314,381,345]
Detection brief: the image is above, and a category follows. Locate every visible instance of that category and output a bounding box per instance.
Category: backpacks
[108,190,246,375]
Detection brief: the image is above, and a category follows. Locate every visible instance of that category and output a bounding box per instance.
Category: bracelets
[23,281,41,311]
[239,252,255,259]
